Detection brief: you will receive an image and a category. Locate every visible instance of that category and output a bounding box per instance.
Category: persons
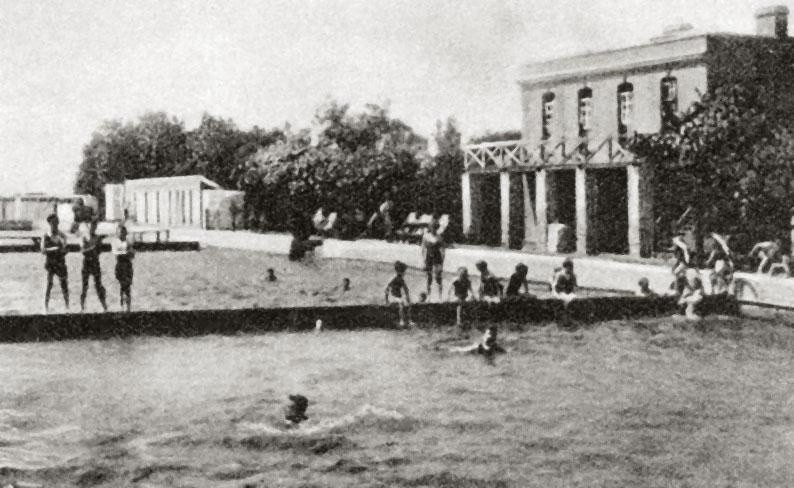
[442,326,504,355]
[40,213,68,311]
[285,394,308,425]
[79,222,109,311]
[265,268,277,281]
[639,223,792,325]
[385,218,578,326]
[343,278,351,291]
[111,227,134,311]
